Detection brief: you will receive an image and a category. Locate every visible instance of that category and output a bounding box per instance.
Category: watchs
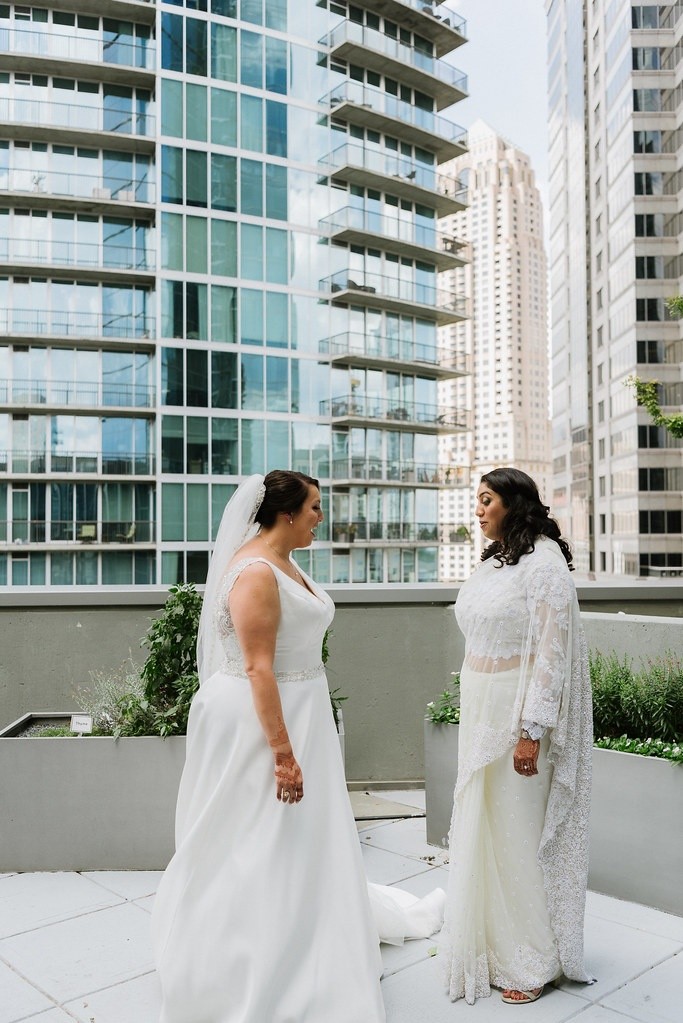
[521,730,531,741]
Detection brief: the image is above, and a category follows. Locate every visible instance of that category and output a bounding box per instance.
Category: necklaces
[256,533,298,575]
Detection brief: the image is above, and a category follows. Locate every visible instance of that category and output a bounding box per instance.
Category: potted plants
[348,525,356,542]
[335,525,346,542]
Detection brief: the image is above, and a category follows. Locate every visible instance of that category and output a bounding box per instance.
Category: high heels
[502,976,561,1004]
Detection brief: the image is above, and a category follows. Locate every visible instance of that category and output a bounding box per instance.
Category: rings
[522,765,530,769]
[283,792,290,799]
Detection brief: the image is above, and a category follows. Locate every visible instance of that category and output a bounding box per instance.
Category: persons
[444,468,595,1006]
[152,469,440,1023]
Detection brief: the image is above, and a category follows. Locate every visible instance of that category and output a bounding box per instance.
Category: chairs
[117,523,136,544]
[77,525,95,545]
